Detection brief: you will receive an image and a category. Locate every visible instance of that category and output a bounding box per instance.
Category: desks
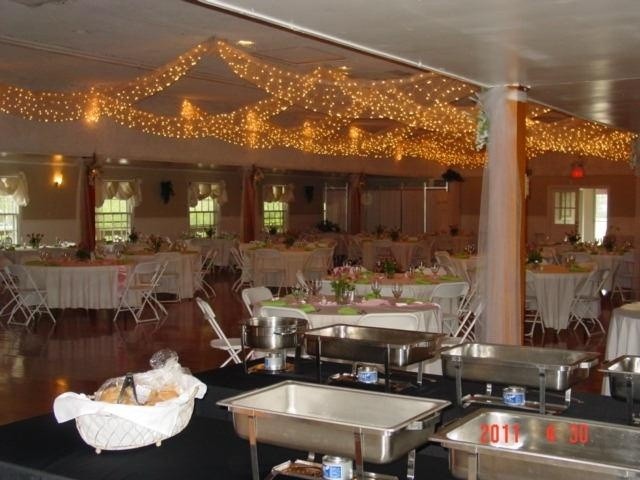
[1,355,640,479]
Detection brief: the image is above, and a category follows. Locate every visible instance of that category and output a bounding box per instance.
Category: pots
[236,315,309,349]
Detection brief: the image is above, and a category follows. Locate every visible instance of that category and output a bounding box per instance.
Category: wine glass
[583,236,605,251]
[34,250,122,265]
[337,258,443,281]
[534,253,576,272]
[289,276,405,309]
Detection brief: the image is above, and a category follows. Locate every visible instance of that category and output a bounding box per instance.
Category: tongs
[117,372,142,407]
